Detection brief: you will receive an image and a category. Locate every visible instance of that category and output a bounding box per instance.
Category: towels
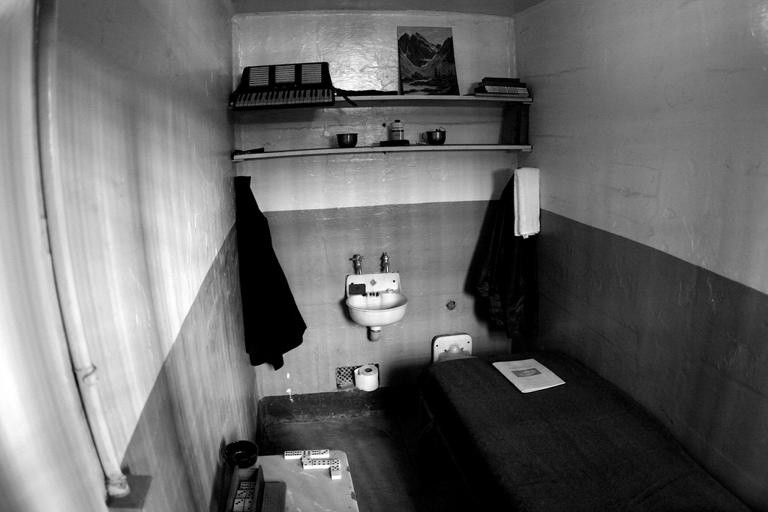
[513,168,541,242]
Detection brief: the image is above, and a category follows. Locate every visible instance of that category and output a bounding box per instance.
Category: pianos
[227,60,335,112]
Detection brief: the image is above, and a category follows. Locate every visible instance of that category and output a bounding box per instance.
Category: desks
[223,447,358,512]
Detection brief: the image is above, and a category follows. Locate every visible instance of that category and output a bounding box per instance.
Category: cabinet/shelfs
[227,97,533,160]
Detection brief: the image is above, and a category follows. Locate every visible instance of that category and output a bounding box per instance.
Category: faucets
[353,255,363,275]
[383,250,390,273]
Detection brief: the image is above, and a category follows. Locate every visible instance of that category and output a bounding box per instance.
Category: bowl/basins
[336,133,357,148]
[422,128,445,146]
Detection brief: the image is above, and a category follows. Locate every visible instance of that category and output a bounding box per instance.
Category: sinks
[345,273,408,326]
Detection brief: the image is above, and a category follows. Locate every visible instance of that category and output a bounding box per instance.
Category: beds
[414,356,756,510]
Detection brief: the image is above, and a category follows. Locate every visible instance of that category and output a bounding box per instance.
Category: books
[492,358,566,395]
